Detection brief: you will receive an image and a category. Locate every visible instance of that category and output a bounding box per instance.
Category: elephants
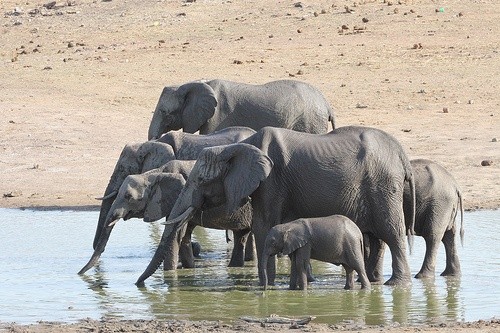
[77,78,464,285]
[261,215,371,292]
